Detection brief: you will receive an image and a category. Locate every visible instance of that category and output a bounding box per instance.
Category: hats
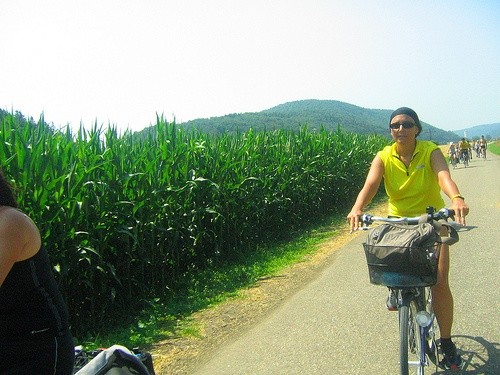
[390,107,419,124]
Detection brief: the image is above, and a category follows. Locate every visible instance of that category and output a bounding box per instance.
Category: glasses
[390,122,418,129]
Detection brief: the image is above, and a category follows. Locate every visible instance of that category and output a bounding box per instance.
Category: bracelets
[451,194,464,201]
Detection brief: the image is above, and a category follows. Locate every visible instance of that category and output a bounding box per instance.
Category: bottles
[416,310,432,327]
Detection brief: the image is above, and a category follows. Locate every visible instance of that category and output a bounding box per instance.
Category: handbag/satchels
[366,222,442,275]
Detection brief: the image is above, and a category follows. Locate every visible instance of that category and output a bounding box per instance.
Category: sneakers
[441,342,463,370]
[385,290,399,311]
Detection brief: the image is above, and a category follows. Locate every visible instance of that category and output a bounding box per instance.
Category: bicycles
[449,146,486,170]
[356,208,457,375]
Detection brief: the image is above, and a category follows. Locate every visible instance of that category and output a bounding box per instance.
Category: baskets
[363,243,441,288]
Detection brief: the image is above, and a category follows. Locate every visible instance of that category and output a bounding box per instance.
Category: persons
[344,107,469,371]
[448,141,459,163]
[0,167,76,375]
[456,135,487,159]
[459,138,470,162]
